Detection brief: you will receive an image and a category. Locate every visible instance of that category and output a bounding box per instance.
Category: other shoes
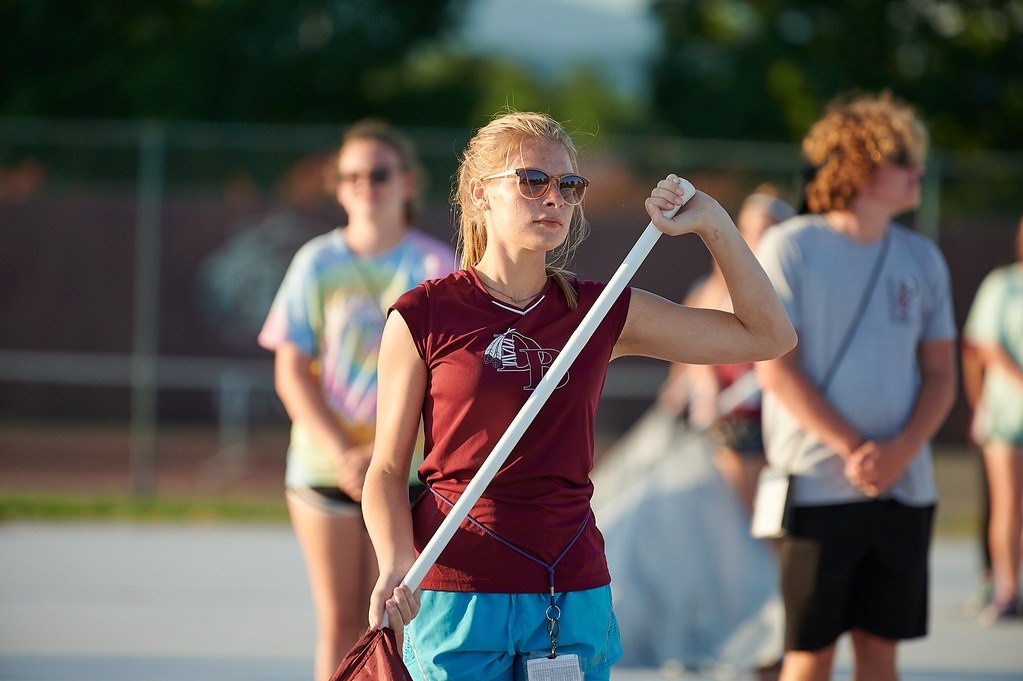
[982,592,1023,624]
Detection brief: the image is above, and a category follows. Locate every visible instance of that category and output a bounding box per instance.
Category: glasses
[477,169,590,206]
[888,146,911,167]
[335,166,409,185]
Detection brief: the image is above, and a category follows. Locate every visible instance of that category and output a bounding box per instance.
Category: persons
[258,120,457,681]
[361,111,799,681]
[760,98,958,680]
[961,220,1023,622]
[661,184,799,519]
